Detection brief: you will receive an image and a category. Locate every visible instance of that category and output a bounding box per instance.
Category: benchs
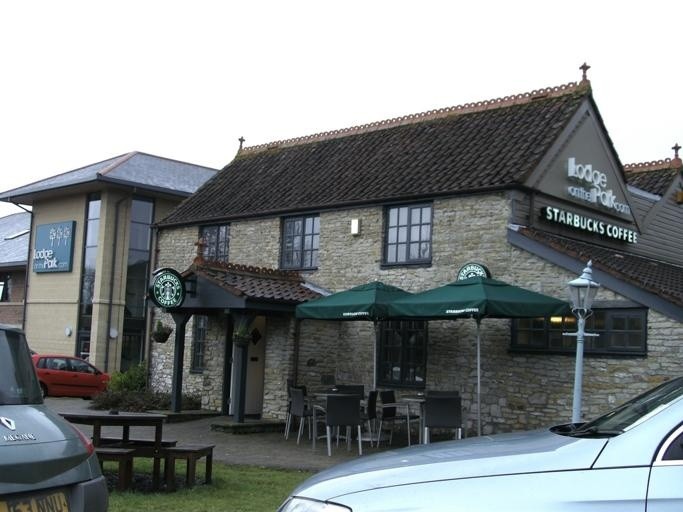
[90,436,177,487]
[94,448,137,490]
[163,443,215,488]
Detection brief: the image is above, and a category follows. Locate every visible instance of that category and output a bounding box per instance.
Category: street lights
[559,258,605,423]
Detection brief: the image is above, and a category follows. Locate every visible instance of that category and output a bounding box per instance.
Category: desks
[57,411,167,489]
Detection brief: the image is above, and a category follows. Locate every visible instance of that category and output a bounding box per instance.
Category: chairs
[284,385,461,457]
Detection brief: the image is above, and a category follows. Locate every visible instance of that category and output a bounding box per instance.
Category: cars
[0,325,108,512]
[275,373,683,512]
[26,345,112,400]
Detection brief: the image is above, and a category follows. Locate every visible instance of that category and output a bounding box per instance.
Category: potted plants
[153,321,174,342]
[233,317,252,347]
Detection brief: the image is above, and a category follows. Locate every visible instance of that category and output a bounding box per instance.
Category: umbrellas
[295,281,414,392]
[388,276,573,435]
